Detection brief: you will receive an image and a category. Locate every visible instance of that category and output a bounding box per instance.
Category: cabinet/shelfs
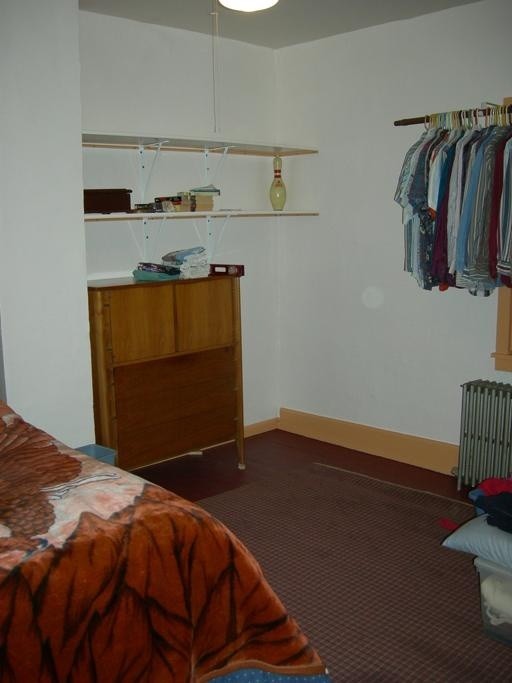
[81,129,319,222]
[87,275,246,471]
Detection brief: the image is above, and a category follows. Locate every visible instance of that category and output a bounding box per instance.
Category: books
[132,183,221,212]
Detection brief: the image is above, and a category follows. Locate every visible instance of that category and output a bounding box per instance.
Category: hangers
[405,104,512,153]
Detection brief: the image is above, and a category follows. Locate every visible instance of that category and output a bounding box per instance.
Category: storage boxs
[472,556,512,645]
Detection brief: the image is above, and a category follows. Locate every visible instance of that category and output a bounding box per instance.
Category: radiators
[455,379,512,492]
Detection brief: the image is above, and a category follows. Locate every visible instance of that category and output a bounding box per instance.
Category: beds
[0,401,234,682]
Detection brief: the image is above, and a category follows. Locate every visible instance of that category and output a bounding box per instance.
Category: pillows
[440,514,511,570]
